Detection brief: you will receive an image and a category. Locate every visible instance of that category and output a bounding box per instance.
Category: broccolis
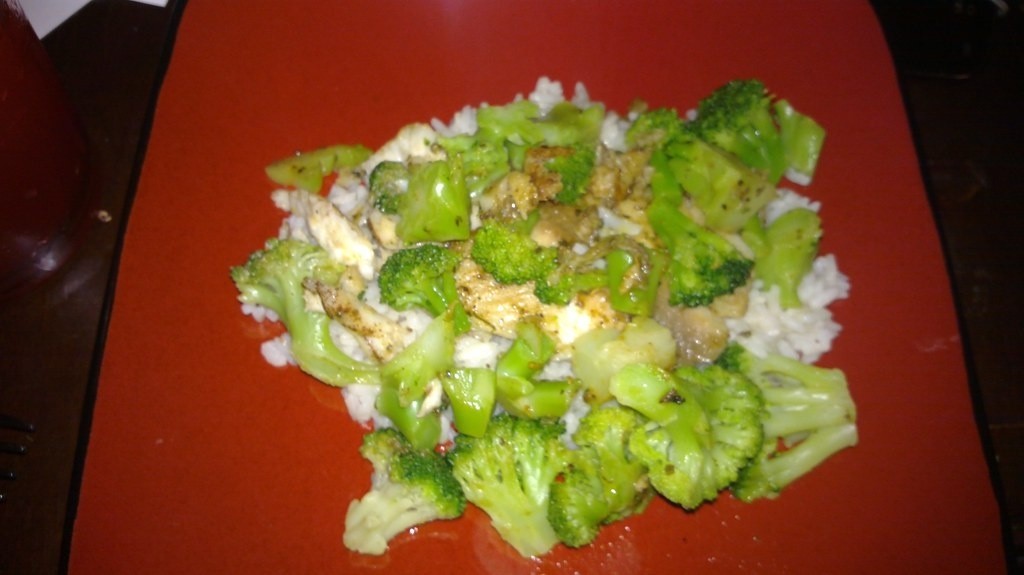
[231,72,859,561]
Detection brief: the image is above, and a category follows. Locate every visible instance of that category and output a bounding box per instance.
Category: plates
[70,0,1008,575]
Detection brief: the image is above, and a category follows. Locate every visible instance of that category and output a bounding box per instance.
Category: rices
[238,77,849,458]
